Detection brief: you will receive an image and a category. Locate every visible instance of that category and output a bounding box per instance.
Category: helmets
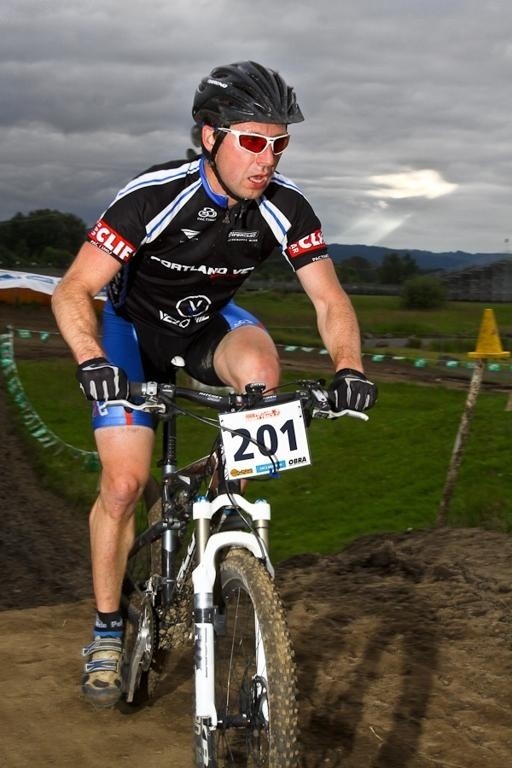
[191,60,305,127]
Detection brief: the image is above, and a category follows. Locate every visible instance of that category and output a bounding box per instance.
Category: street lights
[468,308,512,359]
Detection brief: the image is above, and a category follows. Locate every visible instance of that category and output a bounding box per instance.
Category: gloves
[328,367,378,413]
[75,357,131,403]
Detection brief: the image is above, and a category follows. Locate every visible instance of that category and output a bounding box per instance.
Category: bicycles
[98,377,369,768]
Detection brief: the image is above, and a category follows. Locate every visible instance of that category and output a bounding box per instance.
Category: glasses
[214,127,291,156]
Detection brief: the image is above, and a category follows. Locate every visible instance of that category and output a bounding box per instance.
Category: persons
[47,58,379,711]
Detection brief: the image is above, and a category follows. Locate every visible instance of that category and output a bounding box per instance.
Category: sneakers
[80,633,130,706]
